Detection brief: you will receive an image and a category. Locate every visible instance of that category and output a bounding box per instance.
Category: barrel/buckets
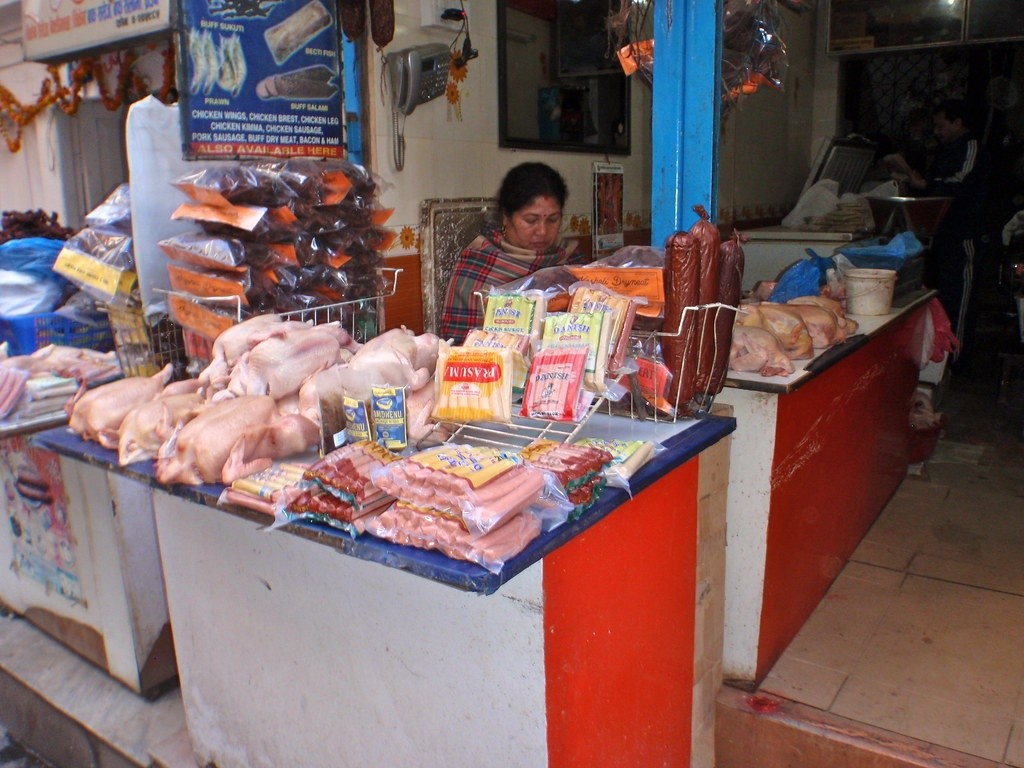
[837,268,897,316]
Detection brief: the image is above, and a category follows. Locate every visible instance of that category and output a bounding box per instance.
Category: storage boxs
[829,36,879,50]
[907,420,943,463]
[830,11,873,38]
[0,311,116,357]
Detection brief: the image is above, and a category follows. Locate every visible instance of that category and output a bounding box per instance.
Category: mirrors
[495,1,632,156]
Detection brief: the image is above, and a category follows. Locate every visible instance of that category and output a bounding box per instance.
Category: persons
[890,99,989,378]
[440,159,584,346]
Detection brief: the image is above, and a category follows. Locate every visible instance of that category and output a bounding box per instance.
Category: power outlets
[420,0,453,30]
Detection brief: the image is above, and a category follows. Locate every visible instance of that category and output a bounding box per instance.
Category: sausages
[433,283,636,420]
[0,340,122,422]
[224,438,655,568]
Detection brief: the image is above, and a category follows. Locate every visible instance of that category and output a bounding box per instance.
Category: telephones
[386,42,454,116]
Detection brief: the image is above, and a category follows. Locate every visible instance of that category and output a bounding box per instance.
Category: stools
[993,345,1024,430]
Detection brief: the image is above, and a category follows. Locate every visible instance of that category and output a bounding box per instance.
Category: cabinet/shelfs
[707,288,937,695]
[0,409,182,703]
[824,0,1024,59]
[27,394,737,768]
